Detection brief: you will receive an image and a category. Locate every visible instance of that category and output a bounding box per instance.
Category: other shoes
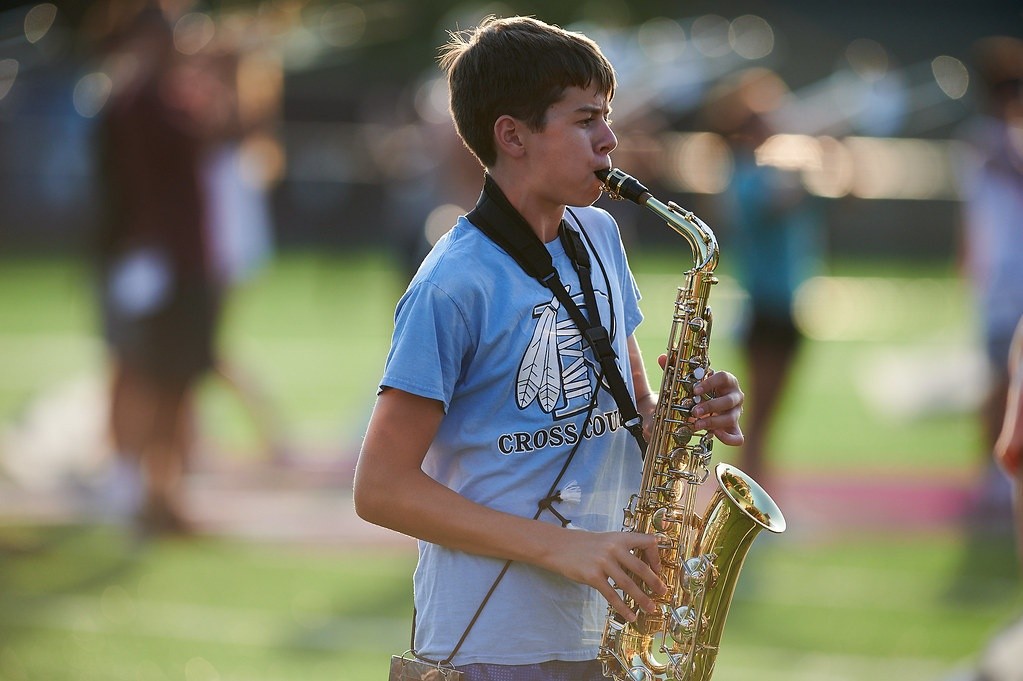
[138,492,193,538]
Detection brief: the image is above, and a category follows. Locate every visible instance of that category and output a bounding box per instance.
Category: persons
[711,110,809,481]
[958,97,1023,481]
[354,16,746,681]
[91,17,220,535]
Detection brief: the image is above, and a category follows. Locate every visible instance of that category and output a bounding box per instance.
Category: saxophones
[595,168,787,679]
